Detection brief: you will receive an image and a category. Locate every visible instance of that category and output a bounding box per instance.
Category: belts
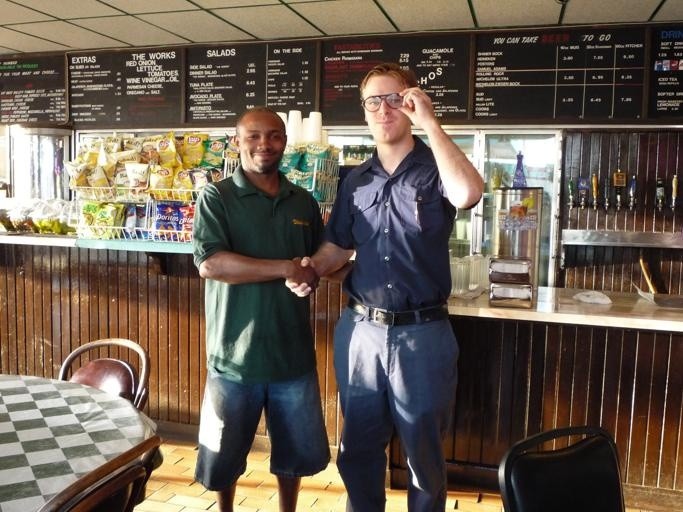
[345,294,449,327]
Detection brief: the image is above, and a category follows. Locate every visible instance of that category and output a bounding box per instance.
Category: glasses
[359,92,405,113]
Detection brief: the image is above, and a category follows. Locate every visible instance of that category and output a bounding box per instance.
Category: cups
[446,253,497,298]
[490,166,504,188]
[275,107,329,146]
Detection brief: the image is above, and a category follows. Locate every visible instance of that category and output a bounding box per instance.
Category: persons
[193,104,355,512]
[285,62,484,512]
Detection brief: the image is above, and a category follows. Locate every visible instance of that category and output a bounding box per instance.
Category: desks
[0,365,159,512]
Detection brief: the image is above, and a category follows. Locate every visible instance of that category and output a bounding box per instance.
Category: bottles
[512,154,527,188]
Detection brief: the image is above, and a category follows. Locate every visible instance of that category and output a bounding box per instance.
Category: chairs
[23,433,161,512]
[491,422,629,512]
[52,333,149,411]
